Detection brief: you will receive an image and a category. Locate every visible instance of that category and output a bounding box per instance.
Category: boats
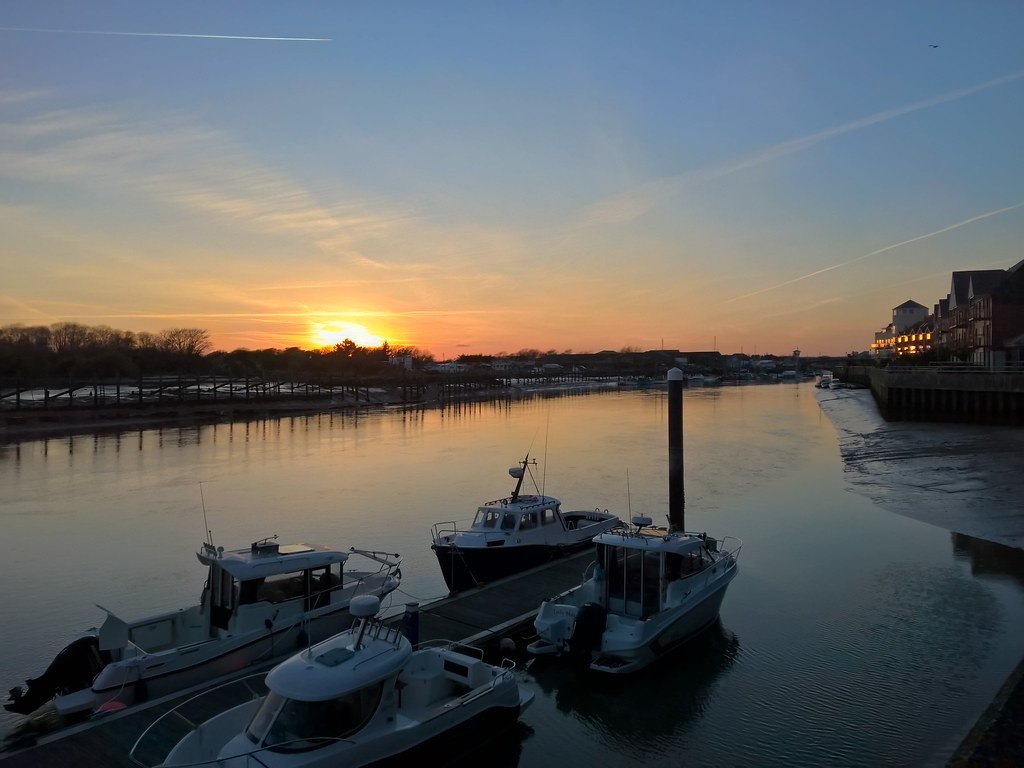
[9,480,402,726]
[130,591,535,768]
[820,375,846,390]
[430,449,628,596]
[528,515,742,679]
